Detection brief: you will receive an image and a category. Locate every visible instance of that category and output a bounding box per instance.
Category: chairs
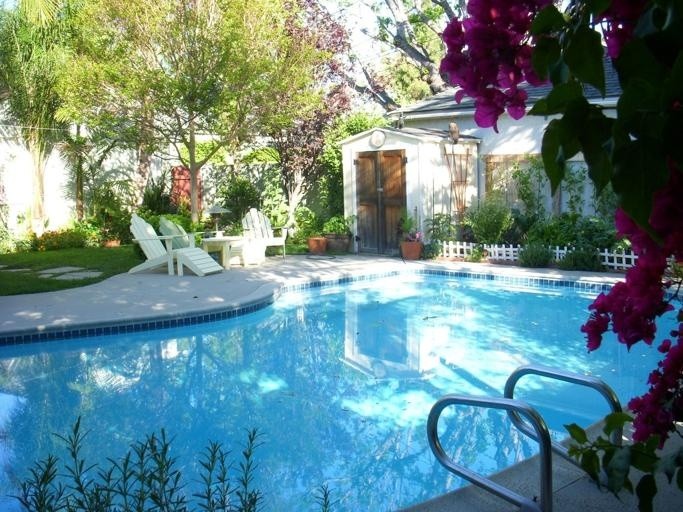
[128,206,288,279]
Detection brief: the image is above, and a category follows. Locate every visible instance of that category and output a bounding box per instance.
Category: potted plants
[308,230,328,255]
[100,231,122,248]
[396,206,425,262]
[323,213,360,254]
